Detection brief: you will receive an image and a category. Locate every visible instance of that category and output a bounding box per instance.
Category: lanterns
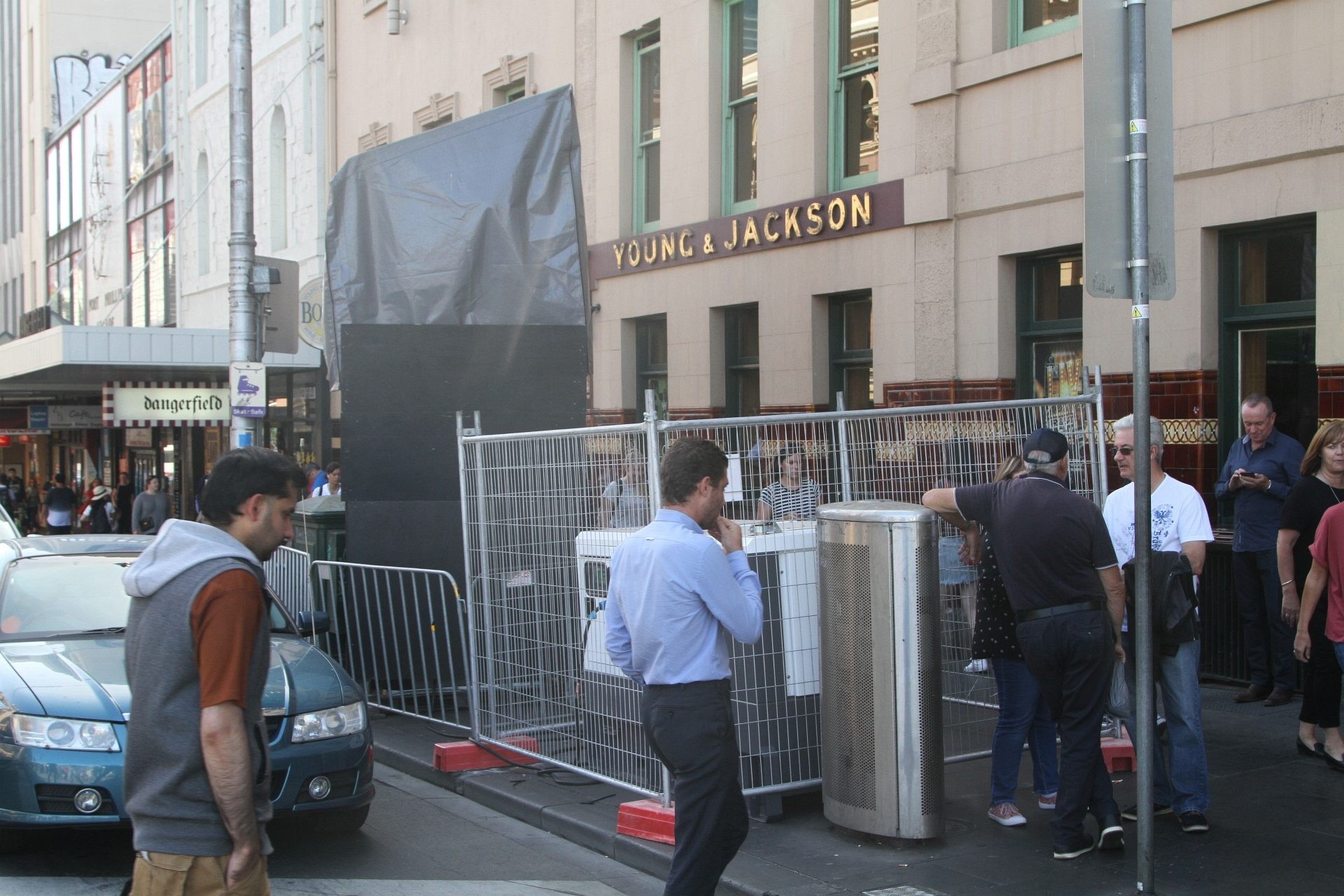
[0,435,11,447]
[17,434,32,444]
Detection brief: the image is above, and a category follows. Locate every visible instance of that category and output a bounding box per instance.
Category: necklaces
[1321,471,1344,504]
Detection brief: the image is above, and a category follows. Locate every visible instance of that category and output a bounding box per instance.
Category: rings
[1293,649,1296,657]
[1284,612,1292,618]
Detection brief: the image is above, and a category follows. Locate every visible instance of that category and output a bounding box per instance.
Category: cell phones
[1240,472,1256,479]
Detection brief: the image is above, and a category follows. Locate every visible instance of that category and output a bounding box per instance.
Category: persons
[596,449,651,529]
[970,454,1063,826]
[758,443,824,523]
[121,445,307,895]
[1216,394,1344,772]
[0,459,349,544]
[1098,413,1212,833]
[603,437,763,896]
[921,427,1125,859]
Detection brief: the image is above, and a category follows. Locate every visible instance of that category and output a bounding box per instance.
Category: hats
[1023,428,1068,465]
[305,462,320,478]
[91,485,112,500]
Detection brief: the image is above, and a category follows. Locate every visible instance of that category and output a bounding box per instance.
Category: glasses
[1110,448,1134,457]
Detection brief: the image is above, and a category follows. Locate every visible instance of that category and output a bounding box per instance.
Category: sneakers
[988,802,1027,826]
[1038,793,1057,809]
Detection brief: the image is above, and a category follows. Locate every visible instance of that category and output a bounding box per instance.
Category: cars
[0,503,376,856]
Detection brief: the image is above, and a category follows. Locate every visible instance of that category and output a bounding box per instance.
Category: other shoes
[1233,683,1272,701]
[1098,815,1125,850]
[1178,810,1209,831]
[1053,833,1095,859]
[1265,688,1292,706]
[1120,802,1174,820]
[963,659,989,672]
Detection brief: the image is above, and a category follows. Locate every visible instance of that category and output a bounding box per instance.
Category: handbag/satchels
[1108,661,1131,718]
[139,517,154,532]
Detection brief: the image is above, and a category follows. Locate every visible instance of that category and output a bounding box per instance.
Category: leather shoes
[1297,736,1325,757]
[1324,752,1344,772]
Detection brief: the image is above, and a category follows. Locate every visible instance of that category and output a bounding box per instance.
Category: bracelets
[1264,479,1273,492]
[1281,578,1294,587]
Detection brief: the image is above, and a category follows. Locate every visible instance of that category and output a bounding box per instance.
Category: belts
[1015,601,1105,622]
